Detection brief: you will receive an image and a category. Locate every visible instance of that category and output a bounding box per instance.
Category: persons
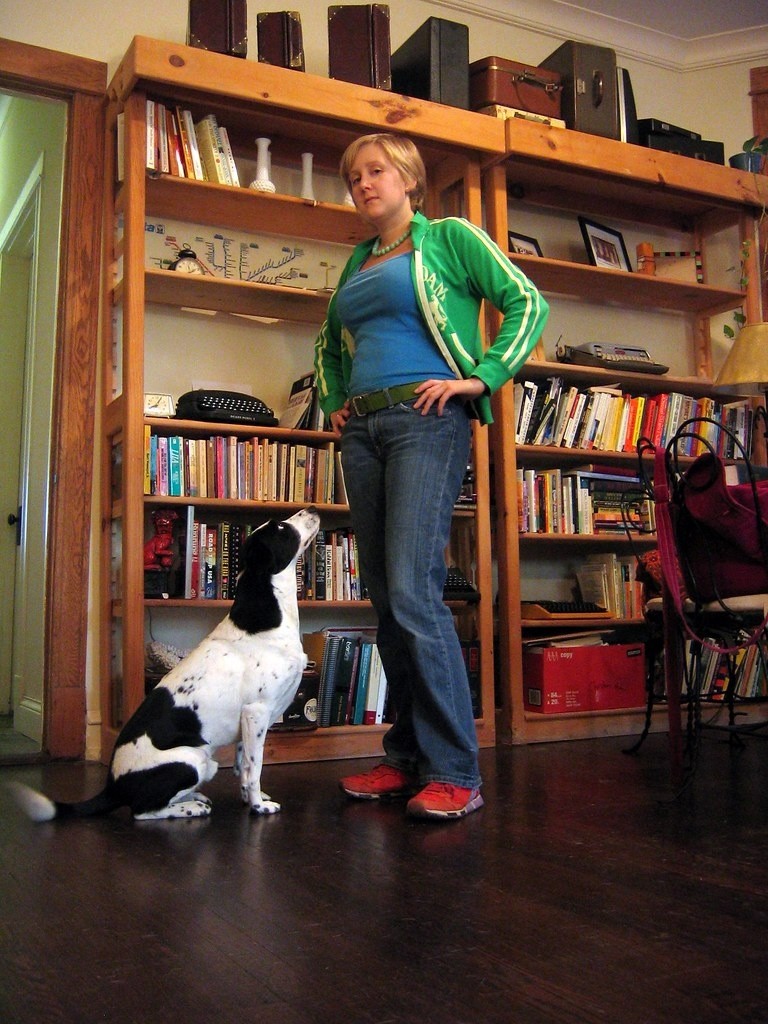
[312,134,549,817]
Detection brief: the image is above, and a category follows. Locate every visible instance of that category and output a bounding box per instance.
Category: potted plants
[728,133,768,174]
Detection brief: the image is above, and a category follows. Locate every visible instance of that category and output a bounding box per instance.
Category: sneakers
[406,782,484,818]
[338,764,421,800]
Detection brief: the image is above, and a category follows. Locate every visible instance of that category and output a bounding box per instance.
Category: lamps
[711,321,768,399]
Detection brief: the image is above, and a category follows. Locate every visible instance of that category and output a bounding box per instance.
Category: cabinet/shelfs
[90,35,768,768]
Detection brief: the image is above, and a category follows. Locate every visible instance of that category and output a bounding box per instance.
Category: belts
[349,381,424,417]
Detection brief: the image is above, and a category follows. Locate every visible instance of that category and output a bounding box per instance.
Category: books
[514,376,752,461]
[578,553,645,619]
[143,425,349,505]
[303,627,388,727]
[143,504,361,602]
[516,463,658,536]
[551,635,612,648]
[146,99,240,187]
[681,637,768,700]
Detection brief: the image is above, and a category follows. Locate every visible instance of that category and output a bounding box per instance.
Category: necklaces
[372,230,410,257]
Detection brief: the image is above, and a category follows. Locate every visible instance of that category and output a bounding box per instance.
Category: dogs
[1,505,321,822]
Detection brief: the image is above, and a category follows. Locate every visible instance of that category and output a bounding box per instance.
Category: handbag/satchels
[653,446,768,652]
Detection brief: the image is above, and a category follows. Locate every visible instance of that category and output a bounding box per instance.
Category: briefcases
[392,16,470,110]
[186,0,247,58]
[538,39,640,144]
[637,118,725,165]
[256,10,305,72]
[327,3,393,91]
[469,56,566,129]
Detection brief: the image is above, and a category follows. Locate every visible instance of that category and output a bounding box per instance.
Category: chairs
[619,415,768,757]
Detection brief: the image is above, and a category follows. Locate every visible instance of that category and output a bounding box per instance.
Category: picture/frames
[577,214,633,273]
[508,229,543,257]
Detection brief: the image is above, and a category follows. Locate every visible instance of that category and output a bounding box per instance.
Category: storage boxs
[188,0,248,57]
[478,104,566,130]
[391,16,468,108]
[256,11,306,72]
[640,117,704,160]
[524,644,646,713]
[328,3,392,91]
